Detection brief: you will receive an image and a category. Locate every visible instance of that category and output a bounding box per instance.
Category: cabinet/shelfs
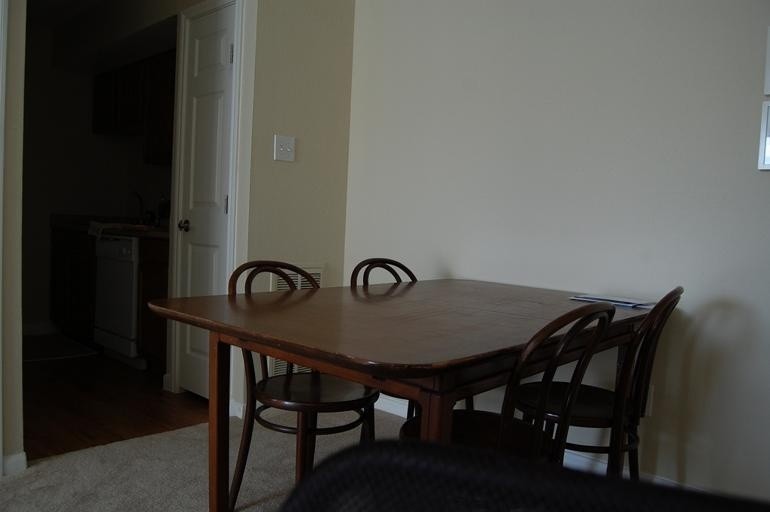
[48,227,169,371]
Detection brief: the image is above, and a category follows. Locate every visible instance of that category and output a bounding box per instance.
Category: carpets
[1,406,407,512]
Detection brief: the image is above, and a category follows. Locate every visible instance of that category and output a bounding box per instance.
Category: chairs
[226,259,381,509]
[399,301,615,501]
[350,257,424,438]
[514,288,685,489]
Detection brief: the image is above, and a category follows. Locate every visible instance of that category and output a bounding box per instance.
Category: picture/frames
[757,101,770,171]
[763,31,770,96]
